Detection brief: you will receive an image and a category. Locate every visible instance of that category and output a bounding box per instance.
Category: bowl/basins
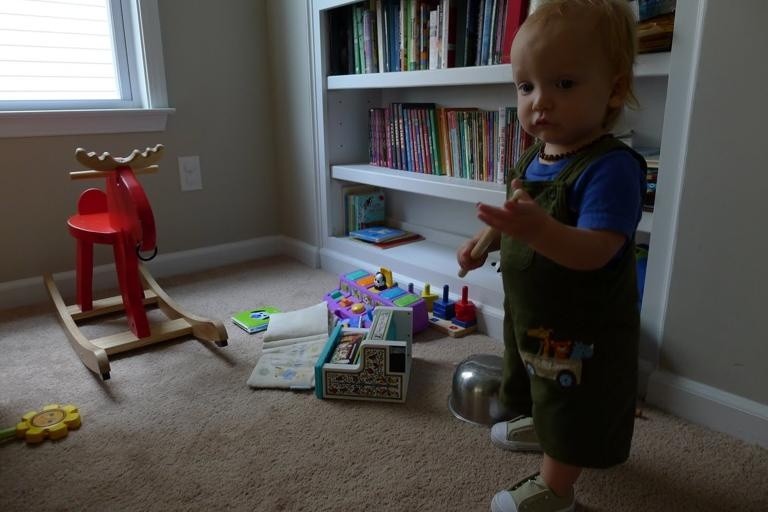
[446,351,507,429]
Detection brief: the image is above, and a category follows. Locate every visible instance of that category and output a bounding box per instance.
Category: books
[247,299,335,391]
[230,306,282,335]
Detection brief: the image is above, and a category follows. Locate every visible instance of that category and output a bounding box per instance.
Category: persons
[456,0,648,511]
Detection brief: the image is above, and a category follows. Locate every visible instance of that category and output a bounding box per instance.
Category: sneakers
[489,466,577,512]
[489,414,546,452]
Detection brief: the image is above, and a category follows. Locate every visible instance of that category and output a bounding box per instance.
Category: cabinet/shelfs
[311,0,677,358]
